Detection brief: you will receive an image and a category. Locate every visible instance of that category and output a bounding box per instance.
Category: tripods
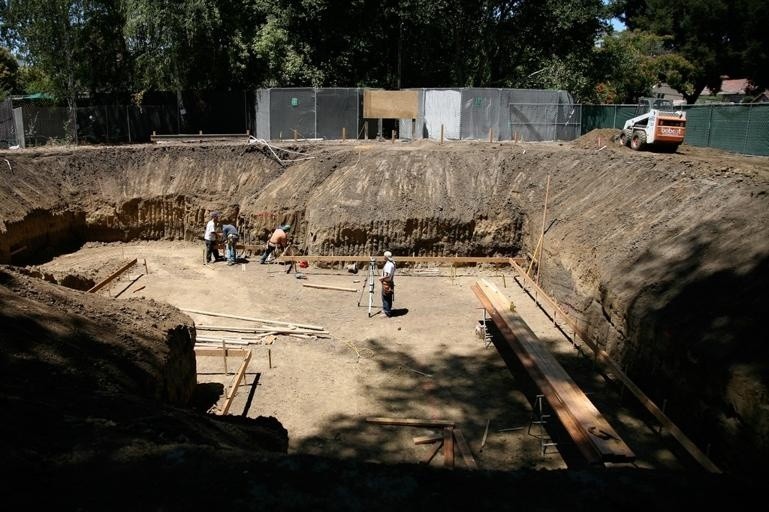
[356,264,381,318]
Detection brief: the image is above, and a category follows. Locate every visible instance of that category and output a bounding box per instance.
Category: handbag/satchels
[383,281,394,294]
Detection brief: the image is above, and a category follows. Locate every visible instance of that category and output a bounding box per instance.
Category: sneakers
[379,310,387,317]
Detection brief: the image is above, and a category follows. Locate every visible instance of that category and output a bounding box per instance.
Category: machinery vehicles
[619,97,688,153]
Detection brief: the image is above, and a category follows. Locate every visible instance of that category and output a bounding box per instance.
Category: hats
[212,212,220,217]
[282,225,290,232]
[384,251,394,262]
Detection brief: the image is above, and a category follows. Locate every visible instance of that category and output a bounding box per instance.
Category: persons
[379,251,395,316]
[259,224,290,264]
[204,213,222,263]
[222,224,239,266]
